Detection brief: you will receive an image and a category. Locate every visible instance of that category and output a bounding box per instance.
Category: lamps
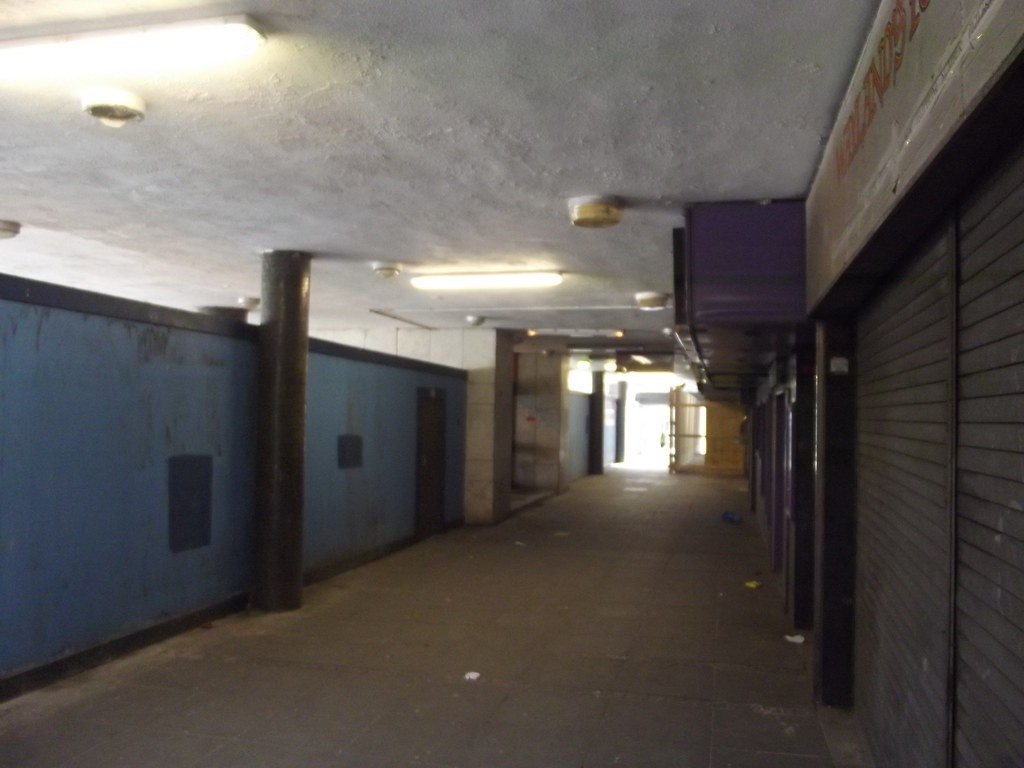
[0,220,21,240]
[238,298,261,309]
[566,194,626,227]
[372,262,403,280]
[635,291,670,311]
[467,316,486,326]
[81,89,147,128]
